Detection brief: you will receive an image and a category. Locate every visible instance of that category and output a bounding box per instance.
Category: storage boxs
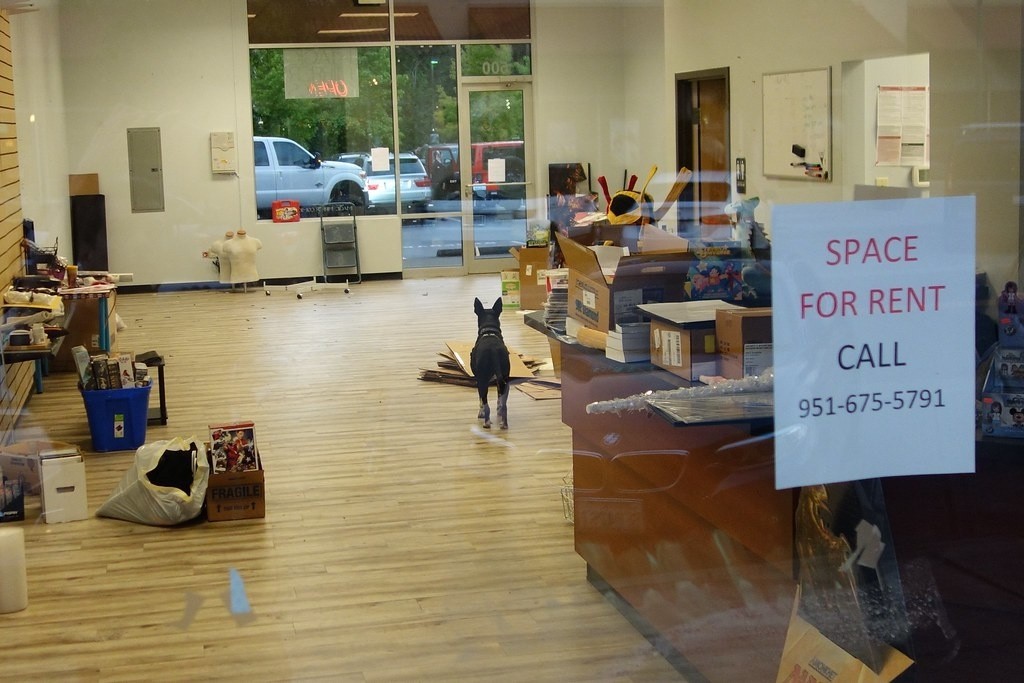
[0,474,25,523]
[48,287,117,373]
[648,306,774,382]
[499,247,556,310]
[979,313,1024,439]
[38,446,87,523]
[0,438,85,495]
[690,238,745,302]
[69,173,99,197]
[556,224,690,334]
[201,421,267,521]
[72,345,153,451]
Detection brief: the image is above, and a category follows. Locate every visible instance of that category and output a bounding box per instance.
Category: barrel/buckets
[78,377,152,453]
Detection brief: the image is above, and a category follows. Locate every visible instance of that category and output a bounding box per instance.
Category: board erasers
[792,143,806,157]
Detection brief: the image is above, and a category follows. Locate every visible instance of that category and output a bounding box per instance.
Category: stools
[146,356,167,426]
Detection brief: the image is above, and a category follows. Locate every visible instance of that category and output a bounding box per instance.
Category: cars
[254,136,369,217]
[420,144,459,167]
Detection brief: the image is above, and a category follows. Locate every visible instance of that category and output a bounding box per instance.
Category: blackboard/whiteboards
[761,65,833,184]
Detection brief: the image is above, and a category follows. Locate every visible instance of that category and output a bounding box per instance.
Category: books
[688,239,744,301]
[606,321,650,363]
[39,448,82,493]
[544,273,567,322]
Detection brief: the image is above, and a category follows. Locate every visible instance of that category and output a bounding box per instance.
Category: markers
[790,162,823,178]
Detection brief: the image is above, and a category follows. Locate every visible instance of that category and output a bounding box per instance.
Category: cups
[67,266,78,288]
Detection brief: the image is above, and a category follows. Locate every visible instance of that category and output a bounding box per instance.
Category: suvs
[338,151,432,222]
[426,140,526,215]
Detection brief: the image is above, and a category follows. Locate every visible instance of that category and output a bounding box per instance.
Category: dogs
[470,297,511,429]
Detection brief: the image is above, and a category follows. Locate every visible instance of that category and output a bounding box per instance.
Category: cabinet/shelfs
[0,277,78,365]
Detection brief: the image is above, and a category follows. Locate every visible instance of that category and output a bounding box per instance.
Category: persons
[1002,281,1022,314]
[223,231,261,283]
[210,231,235,283]
